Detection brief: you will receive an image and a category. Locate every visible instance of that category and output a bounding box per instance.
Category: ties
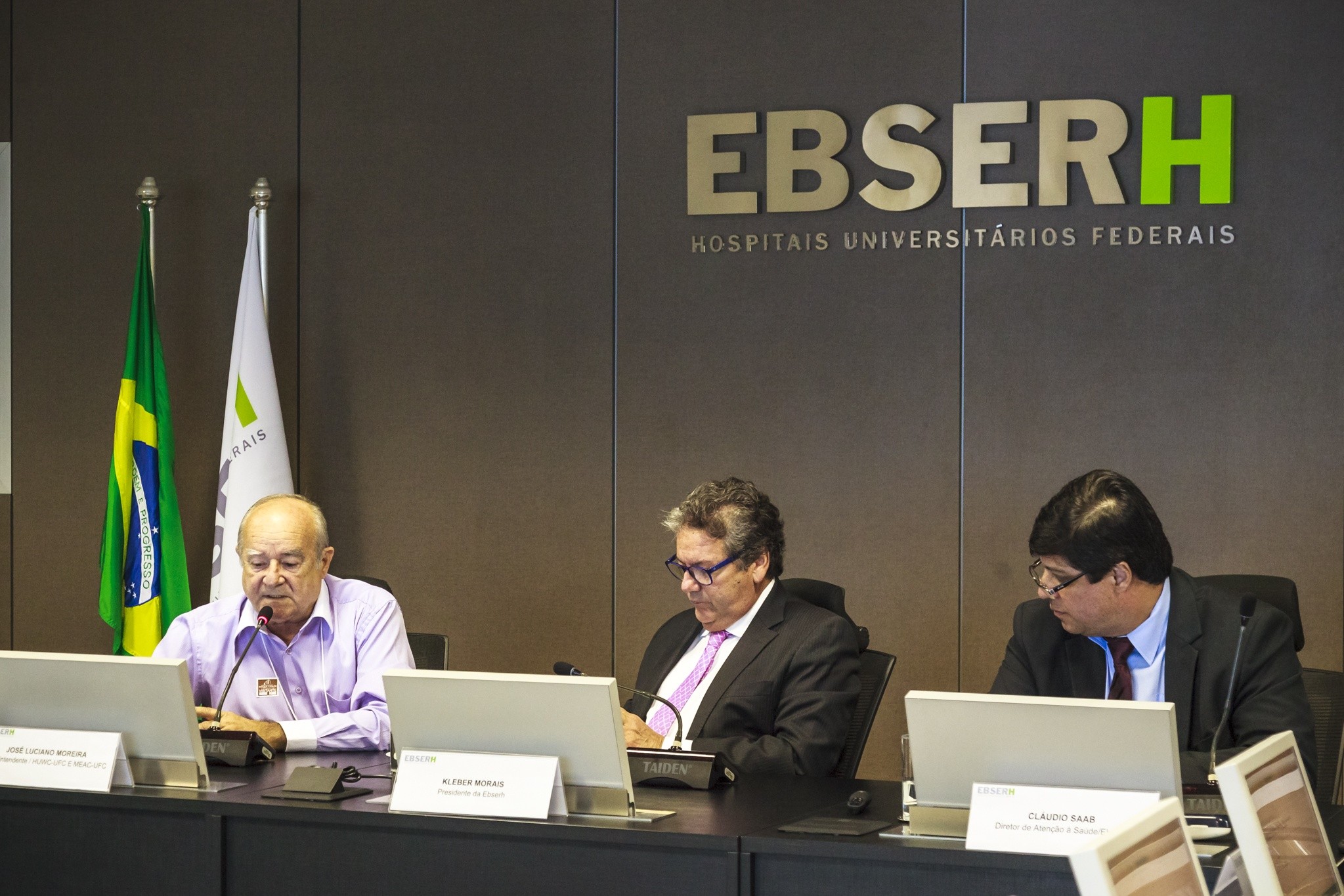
[1103,636,1134,700]
[647,629,734,737]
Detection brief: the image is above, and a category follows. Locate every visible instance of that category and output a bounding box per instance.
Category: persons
[153,494,415,754]
[620,477,862,777]
[984,468,1319,801]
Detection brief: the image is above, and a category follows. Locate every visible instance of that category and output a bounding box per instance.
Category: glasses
[1029,558,1090,598]
[665,552,742,581]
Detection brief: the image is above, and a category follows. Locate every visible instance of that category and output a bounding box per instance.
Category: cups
[390,730,398,772]
[901,734,916,821]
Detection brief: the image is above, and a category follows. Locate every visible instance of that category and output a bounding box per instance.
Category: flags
[205,204,295,603]
[95,203,193,658]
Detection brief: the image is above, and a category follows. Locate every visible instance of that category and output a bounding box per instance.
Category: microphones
[554,660,734,793]
[1187,589,1256,819]
[201,604,273,770]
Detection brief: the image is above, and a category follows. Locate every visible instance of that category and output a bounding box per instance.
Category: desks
[0,752,1344,896]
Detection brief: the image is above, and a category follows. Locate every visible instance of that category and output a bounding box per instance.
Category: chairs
[1208,575,1344,806]
[341,575,451,670]
[780,578,896,779]
